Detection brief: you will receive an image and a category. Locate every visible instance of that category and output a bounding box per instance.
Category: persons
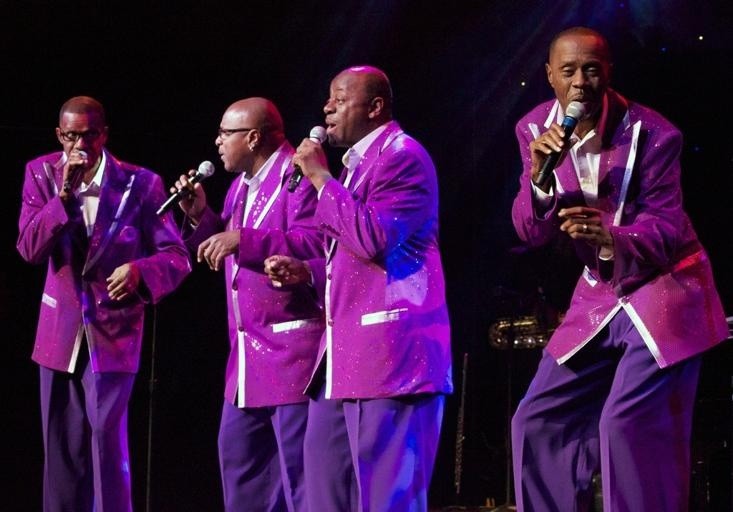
[262,64,455,512]
[171,96,327,509]
[509,23,731,512]
[14,95,195,509]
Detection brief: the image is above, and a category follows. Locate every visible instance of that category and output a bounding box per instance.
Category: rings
[584,224,589,233]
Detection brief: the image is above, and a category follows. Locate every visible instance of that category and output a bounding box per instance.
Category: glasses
[58,128,102,142]
[216,125,251,139]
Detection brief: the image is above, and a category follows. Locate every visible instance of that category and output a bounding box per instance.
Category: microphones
[158,159,216,220]
[534,99,587,192]
[287,126,327,193]
[62,147,88,197]
